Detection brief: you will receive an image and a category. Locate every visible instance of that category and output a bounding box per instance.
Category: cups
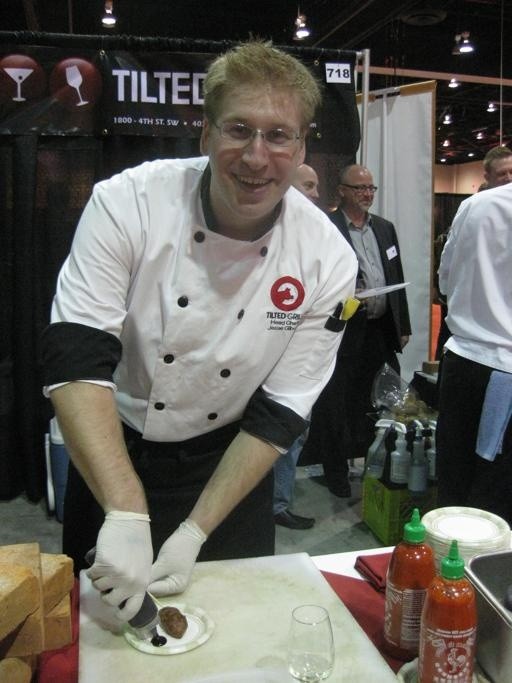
[288,605,335,682]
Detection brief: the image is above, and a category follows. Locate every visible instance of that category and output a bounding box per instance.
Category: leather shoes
[330,479,353,497]
[276,508,315,531]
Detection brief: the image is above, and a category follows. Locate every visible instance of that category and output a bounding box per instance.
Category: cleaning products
[364,419,439,492]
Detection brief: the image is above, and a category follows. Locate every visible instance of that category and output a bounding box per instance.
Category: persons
[434,180,512,529]
[482,143,512,185]
[36,38,361,624]
[273,162,319,529]
[329,162,413,496]
[433,226,452,361]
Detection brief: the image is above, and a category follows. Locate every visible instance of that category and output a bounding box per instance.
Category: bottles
[385,509,438,663]
[416,539,476,683]
[83,545,161,638]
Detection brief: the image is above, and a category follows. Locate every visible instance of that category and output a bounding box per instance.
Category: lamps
[294,5,310,43]
[438,103,505,164]
[453,7,474,56]
[100,0,116,26]
[449,79,459,88]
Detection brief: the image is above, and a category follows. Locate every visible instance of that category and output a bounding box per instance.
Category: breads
[0,542,75,683]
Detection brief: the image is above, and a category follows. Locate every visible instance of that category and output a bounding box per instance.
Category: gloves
[150,518,207,597]
[86,510,154,624]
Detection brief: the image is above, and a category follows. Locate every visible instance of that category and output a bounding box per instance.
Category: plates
[394,656,488,683]
[122,605,215,656]
[354,280,409,299]
[421,504,512,579]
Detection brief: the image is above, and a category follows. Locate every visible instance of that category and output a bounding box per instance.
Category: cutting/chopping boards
[76,551,401,683]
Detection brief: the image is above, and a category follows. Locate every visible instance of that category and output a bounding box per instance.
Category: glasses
[214,123,302,148]
[342,184,377,193]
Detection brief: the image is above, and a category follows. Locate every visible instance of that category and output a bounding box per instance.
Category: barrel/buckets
[420,506,512,572]
